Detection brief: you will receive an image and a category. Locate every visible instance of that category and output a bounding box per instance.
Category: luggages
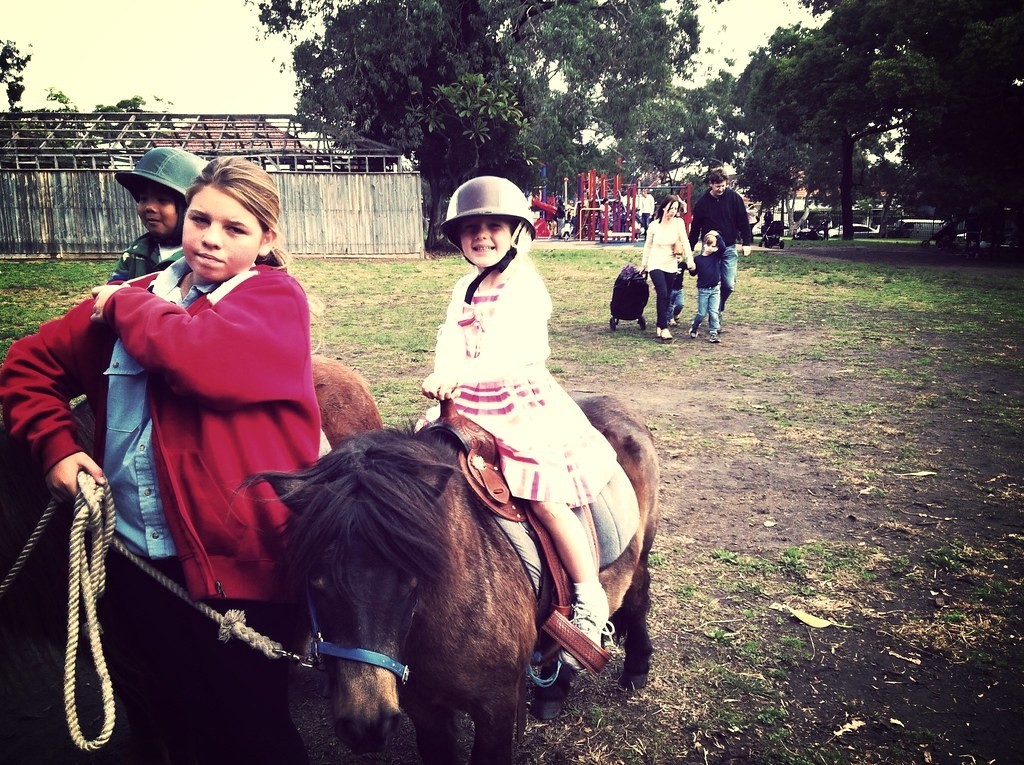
[609,264,649,330]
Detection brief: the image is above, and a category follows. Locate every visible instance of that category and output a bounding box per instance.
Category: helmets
[439,176,536,251]
[115,146,209,204]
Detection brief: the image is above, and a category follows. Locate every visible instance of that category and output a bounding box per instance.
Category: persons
[639,196,694,339]
[689,230,727,343]
[0,156,322,765]
[548,188,774,240]
[107,147,209,282]
[413,177,617,672]
[688,169,754,332]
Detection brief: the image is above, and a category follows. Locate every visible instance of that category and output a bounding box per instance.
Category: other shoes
[668,315,680,328]
[656,326,673,339]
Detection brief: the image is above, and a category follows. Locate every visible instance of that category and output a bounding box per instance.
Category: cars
[827,224,879,237]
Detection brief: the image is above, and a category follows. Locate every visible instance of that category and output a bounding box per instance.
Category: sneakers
[561,581,609,669]
[689,321,698,338]
[709,333,721,343]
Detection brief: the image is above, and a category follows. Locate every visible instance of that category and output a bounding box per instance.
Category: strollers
[921,222,957,250]
[759,220,784,249]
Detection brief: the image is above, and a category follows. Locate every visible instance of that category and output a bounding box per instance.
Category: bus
[793,209,901,230]
[894,219,946,235]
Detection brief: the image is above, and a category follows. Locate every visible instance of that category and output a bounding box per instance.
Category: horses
[0,354,386,723]
[228,393,660,764]
